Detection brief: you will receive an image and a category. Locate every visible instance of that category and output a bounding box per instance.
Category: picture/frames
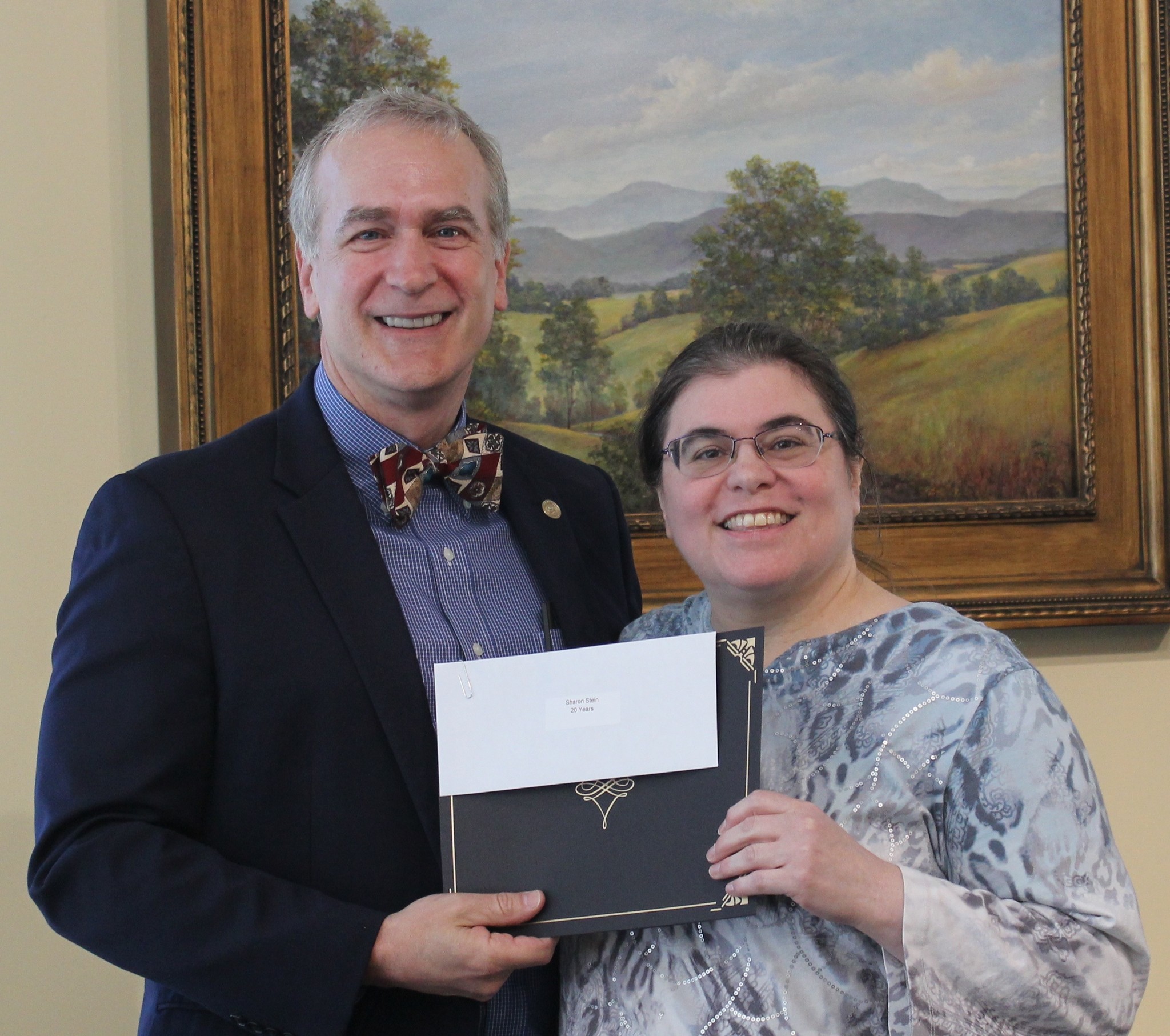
[153,0,1170,633]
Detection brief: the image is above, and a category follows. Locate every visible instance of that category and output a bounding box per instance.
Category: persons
[24,86,642,1036]
[562,323,1151,1036]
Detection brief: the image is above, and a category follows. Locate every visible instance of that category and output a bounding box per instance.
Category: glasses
[660,424,845,479]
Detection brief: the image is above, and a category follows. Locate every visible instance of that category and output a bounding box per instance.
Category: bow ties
[367,433,505,529]
